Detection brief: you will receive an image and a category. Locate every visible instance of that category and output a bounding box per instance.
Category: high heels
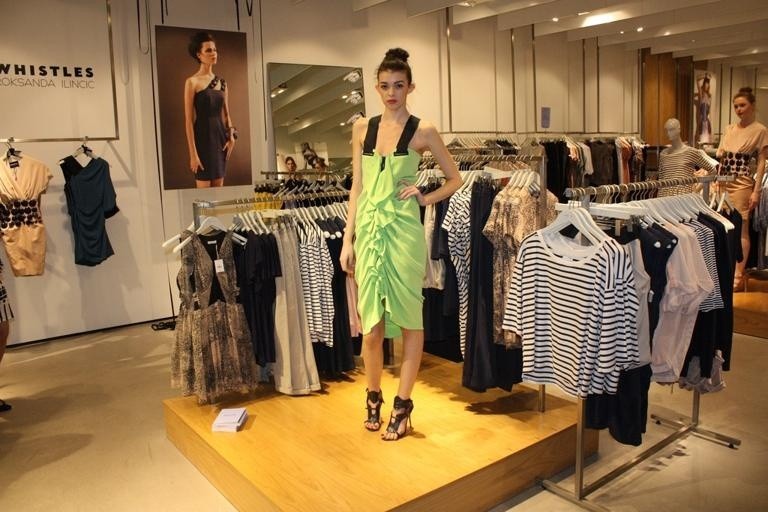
[364,388,385,431]
[383,397,414,440]
[733,271,750,292]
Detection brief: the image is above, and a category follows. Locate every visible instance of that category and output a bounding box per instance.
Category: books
[212,407,247,432]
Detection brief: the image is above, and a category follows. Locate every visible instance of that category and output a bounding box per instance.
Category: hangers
[58,137,111,167]
[160,170,350,254]
[542,178,736,260]
[417,131,650,193]
[1,138,23,161]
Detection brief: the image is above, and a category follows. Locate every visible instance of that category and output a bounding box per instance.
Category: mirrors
[266,63,365,183]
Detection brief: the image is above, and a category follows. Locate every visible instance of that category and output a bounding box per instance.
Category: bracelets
[705,73,707,80]
[225,125,237,140]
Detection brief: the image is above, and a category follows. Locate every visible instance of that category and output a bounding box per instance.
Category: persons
[339,45,462,441]
[696,72,712,143]
[283,156,300,180]
[714,87,768,291]
[656,118,717,200]
[301,141,328,170]
[181,32,238,189]
[316,162,331,181]
